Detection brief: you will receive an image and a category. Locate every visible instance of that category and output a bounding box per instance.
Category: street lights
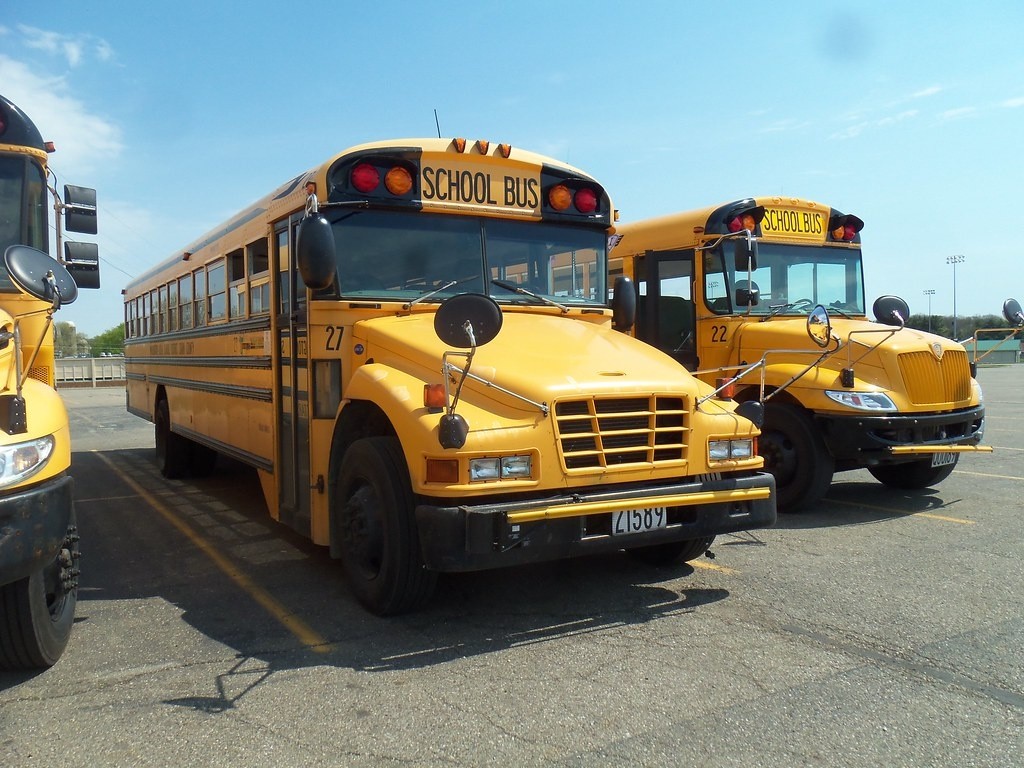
[946,254,966,343]
[922,290,935,333]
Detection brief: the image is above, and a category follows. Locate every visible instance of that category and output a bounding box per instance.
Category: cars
[54,348,124,358]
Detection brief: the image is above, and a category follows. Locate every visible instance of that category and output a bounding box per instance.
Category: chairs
[336,258,774,361]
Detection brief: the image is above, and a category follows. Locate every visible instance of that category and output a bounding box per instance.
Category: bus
[386,196,996,521]
[1,94,100,674]
[120,137,776,617]
